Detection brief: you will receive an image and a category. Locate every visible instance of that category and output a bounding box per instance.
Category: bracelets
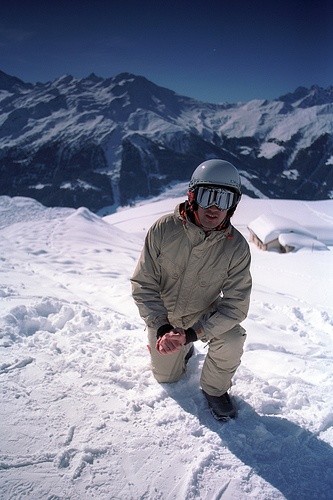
[184,327,198,346]
[156,324,175,339]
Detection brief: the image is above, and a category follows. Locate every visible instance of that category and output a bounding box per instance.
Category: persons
[130,160,253,422]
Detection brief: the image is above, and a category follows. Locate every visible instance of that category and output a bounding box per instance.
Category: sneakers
[201,389,236,421]
[185,345,194,365]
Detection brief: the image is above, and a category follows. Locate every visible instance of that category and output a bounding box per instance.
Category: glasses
[195,186,235,210]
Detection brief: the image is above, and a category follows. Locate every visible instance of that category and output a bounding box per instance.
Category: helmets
[187,159,242,208]
